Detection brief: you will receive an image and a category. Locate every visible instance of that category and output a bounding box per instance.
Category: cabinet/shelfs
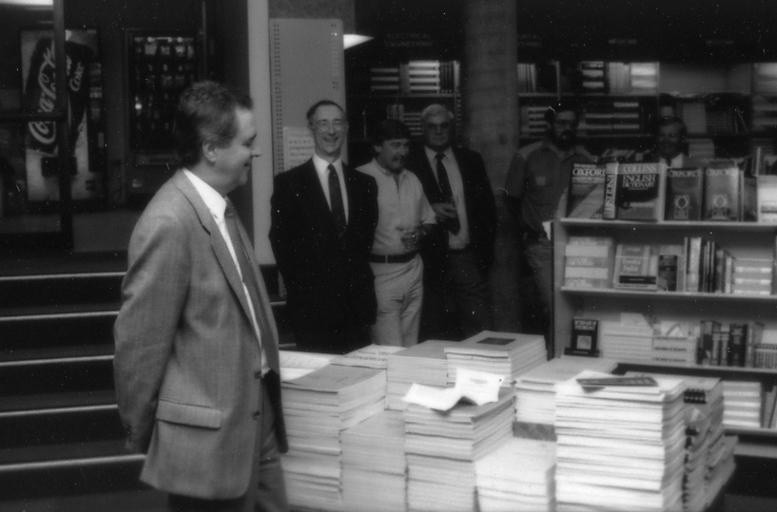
[553,218,774,457]
[359,53,777,173]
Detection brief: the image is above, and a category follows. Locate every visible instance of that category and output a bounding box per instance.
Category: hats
[542,99,582,126]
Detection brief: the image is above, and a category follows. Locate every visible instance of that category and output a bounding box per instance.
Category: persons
[506,100,598,320]
[268,98,379,356]
[405,104,498,341]
[109,79,291,512]
[352,118,439,347]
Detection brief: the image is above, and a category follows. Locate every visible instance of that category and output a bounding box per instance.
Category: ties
[220,196,283,380]
[432,153,460,236]
[324,163,349,251]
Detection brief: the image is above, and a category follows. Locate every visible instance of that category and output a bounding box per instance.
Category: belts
[365,249,423,265]
